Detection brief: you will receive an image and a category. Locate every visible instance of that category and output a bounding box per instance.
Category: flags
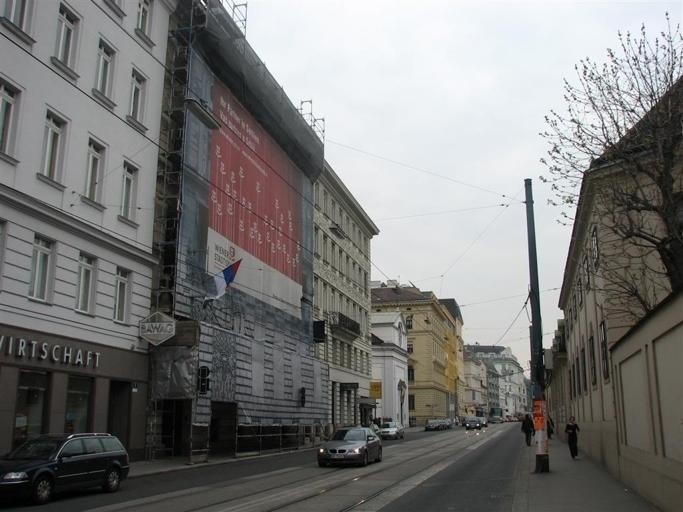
[214,260,243,298]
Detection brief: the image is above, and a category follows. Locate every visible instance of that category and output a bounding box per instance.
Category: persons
[565,416,580,460]
[521,414,554,446]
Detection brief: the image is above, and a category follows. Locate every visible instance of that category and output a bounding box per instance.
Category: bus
[490,407,504,423]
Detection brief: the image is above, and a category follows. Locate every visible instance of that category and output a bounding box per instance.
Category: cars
[317,427,382,467]
[425,418,451,431]
[462,416,488,430]
[379,421,405,440]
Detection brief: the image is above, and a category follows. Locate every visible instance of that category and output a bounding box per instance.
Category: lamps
[132,382,138,393]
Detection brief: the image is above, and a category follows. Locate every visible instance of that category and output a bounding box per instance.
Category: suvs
[1,432,130,504]
